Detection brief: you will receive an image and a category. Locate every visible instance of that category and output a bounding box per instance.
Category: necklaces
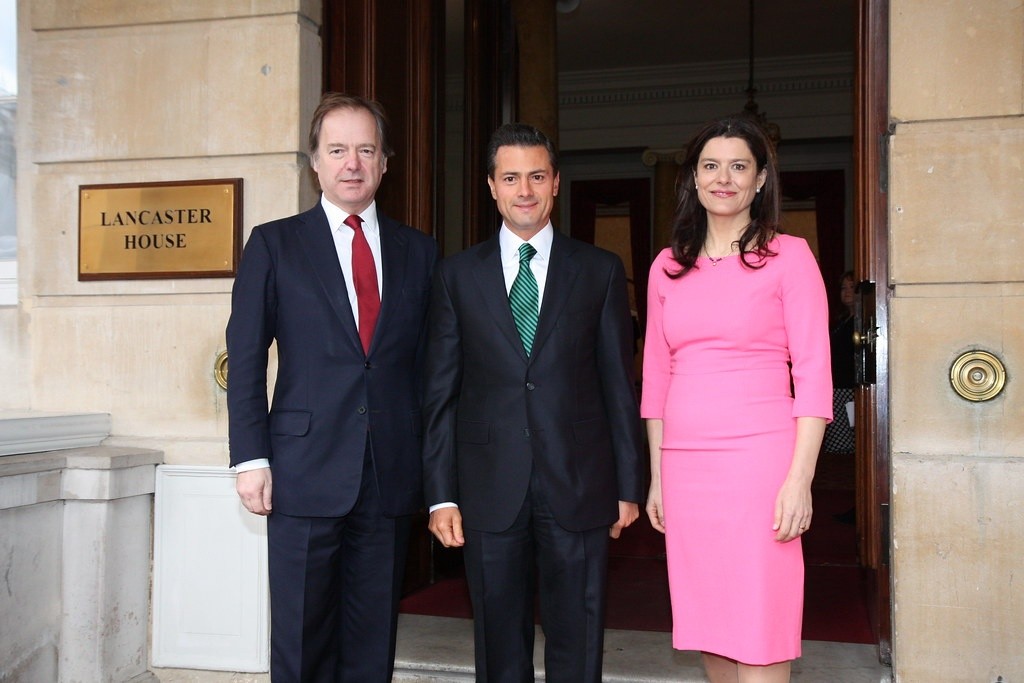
[703,241,739,266]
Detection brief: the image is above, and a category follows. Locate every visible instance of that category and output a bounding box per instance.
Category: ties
[510,243,540,358]
[343,215,382,357]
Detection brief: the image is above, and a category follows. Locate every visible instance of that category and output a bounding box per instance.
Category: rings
[799,526,805,529]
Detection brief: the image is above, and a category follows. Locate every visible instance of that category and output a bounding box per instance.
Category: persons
[421,125,646,683]
[640,120,833,683]
[225,95,441,683]
